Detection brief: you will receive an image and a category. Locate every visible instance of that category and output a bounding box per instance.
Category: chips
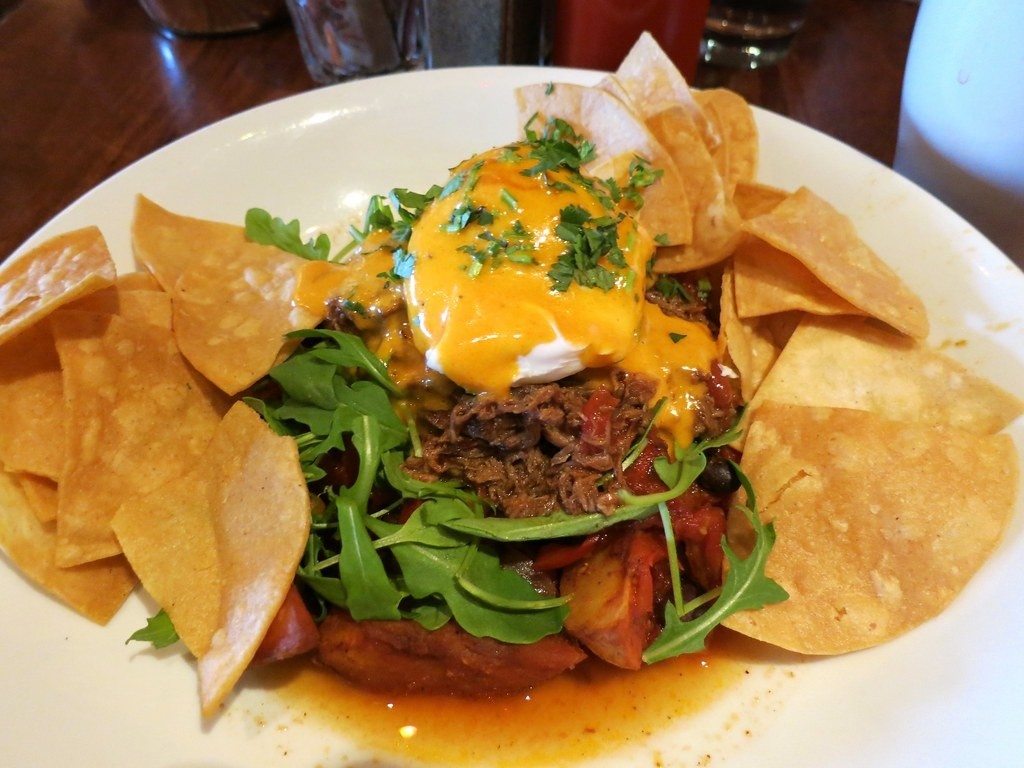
[1,191,359,727]
[511,29,1023,653]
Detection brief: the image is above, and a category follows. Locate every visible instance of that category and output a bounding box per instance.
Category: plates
[0,63,1022,763]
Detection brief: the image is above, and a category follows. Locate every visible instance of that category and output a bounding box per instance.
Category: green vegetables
[120,84,791,665]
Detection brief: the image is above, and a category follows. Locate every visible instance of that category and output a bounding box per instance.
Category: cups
[698,1,810,69]
[892,0,1024,263]
[286,0,508,88]
